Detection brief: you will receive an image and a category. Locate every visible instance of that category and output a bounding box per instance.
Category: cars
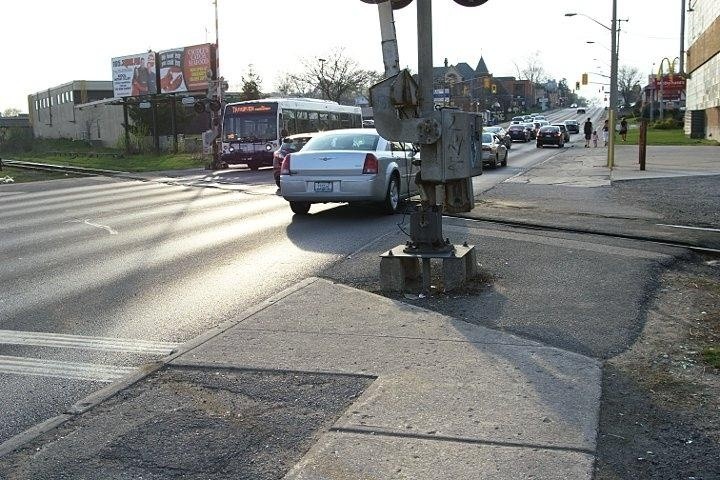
[508,114,551,141]
[280,127,420,213]
[274,132,316,185]
[537,119,580,148]
[482,126,512,166]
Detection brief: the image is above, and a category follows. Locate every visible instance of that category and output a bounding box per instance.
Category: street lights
[565,13,618,167]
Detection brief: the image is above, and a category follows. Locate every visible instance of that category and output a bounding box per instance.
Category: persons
[592,130,599,148]
[585,117,593,148]
[602,120,610,146]
[619,117,628,142]
[130,58,157,95]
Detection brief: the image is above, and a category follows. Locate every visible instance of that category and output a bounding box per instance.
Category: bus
[219,96,363,167]
